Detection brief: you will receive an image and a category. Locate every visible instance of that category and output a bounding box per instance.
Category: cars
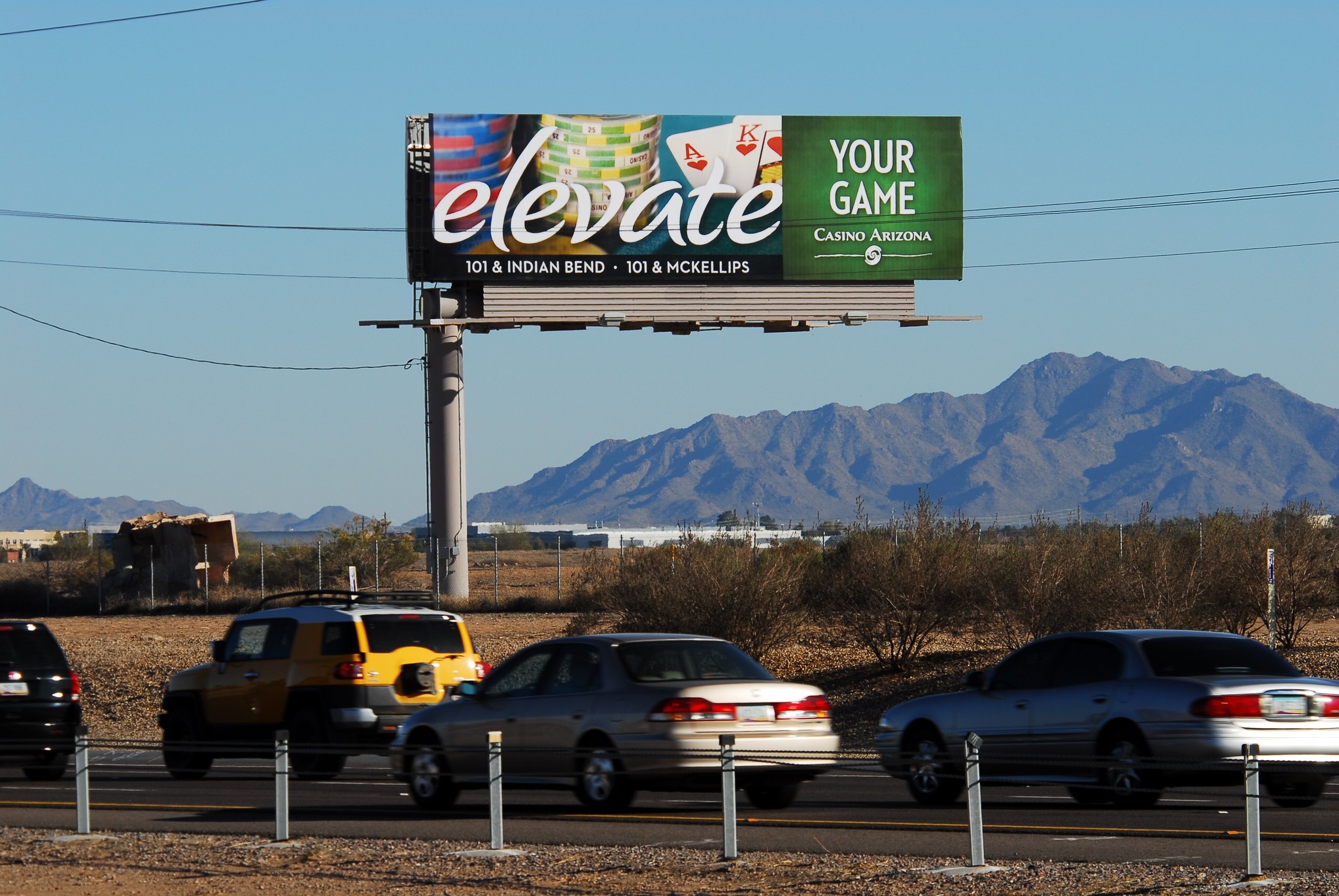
[876,630,1339,807]
[391,631,841,814]
[0,621,81,782]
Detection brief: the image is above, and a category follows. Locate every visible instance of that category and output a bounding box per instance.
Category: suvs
[158,587,492,781]
